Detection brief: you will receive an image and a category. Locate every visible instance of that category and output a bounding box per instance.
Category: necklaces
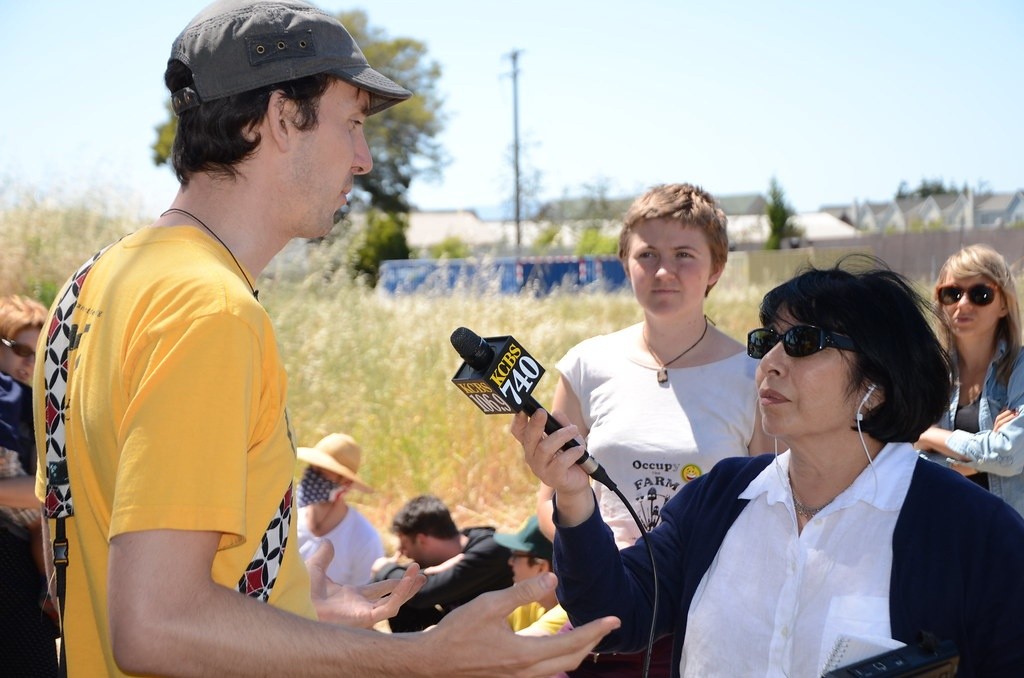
[312,503,333,532]
[641,312,717,384]
[160,205,262,302]
[790,483,839,520]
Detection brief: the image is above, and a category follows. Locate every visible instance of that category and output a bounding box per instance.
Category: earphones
[863,384,876,401]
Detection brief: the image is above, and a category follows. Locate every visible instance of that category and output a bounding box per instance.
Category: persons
[294,429,574,639]
[1,290,70,678]
[905,245,1023,520]
[532,180,788,678]
[508,266,1022,677]
[30,1,624,678]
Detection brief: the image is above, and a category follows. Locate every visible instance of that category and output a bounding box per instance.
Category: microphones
[450,327,618,491]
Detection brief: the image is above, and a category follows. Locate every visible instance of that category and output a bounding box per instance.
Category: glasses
[507,553,528,561]
[0,336,35,358]
[746,324,863,360]
[937,285,995,306]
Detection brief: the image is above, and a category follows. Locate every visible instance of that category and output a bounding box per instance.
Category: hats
[168,0,412,116]
[493,515,552,562]
[297,433,374,495]
